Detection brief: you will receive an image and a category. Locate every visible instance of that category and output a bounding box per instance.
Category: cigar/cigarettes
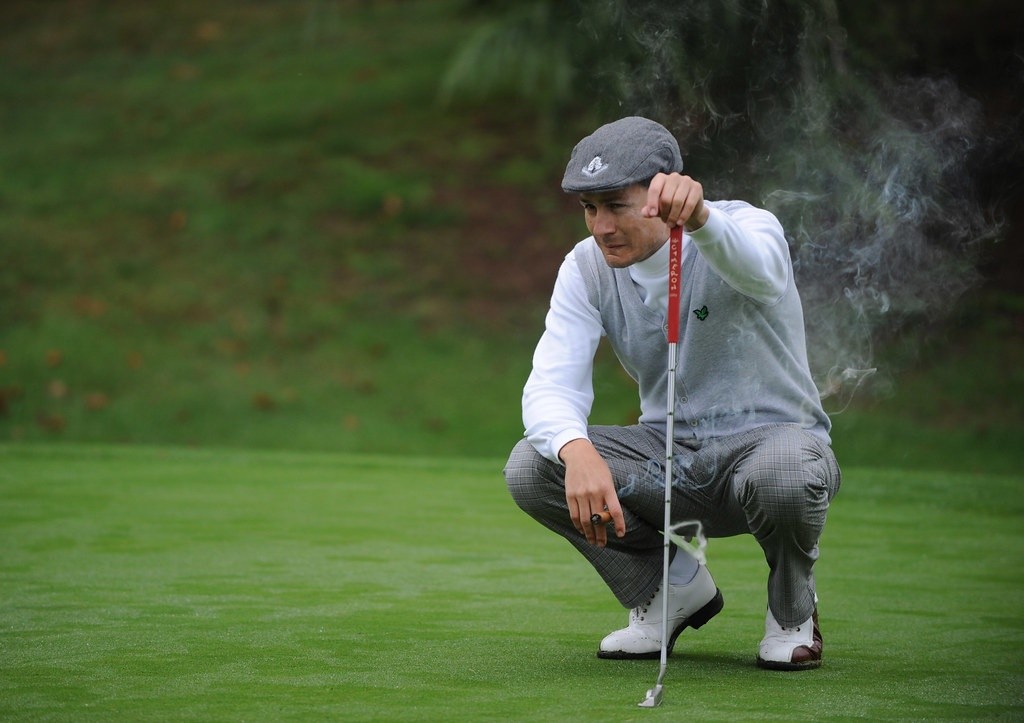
[591,508,614,526]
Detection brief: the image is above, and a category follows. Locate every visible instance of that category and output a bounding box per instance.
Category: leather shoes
[758,592,824,669]
[597,562,726,658]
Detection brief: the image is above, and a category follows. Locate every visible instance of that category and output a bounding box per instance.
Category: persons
[502,117,843,671]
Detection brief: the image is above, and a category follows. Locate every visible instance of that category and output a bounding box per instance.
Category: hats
[560,116,685,195]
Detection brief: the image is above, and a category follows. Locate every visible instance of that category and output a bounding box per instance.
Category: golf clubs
[636,226,682,707]
